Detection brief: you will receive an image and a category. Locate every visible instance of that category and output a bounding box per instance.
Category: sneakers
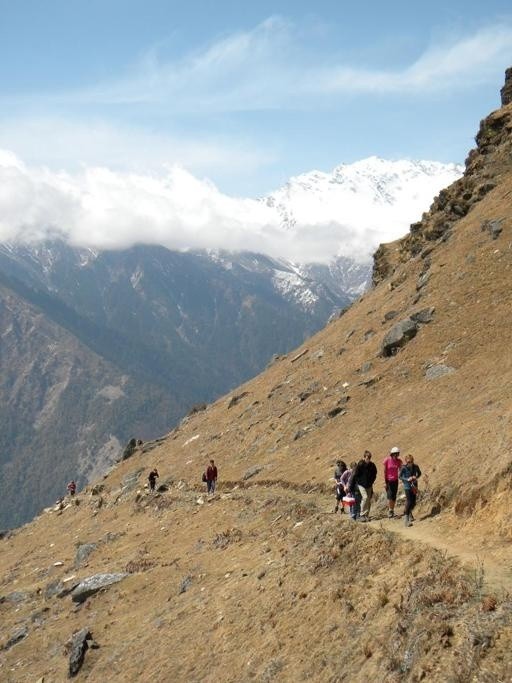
[388,509,398,518]
[340,508,344,512]
[360,514,371,522]
[335,506,339,511]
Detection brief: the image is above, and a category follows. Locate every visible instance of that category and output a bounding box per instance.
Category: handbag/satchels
[202,472,208,482]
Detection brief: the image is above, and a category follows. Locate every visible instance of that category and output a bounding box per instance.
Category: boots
[404,512,414,527]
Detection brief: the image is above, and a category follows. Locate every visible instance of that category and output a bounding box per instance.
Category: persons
[383,447,403,518]
[149,469,160,489]
[206,460,217,493]
[347,450,377,522]
[399,454,421,526]
[340,460,361,520]
[67,480,76,495]
[334,460,349,513]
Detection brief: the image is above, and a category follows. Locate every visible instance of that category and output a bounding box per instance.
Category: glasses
[407,461,413,463]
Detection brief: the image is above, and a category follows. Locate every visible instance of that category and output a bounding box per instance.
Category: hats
[390,447,400,454]
